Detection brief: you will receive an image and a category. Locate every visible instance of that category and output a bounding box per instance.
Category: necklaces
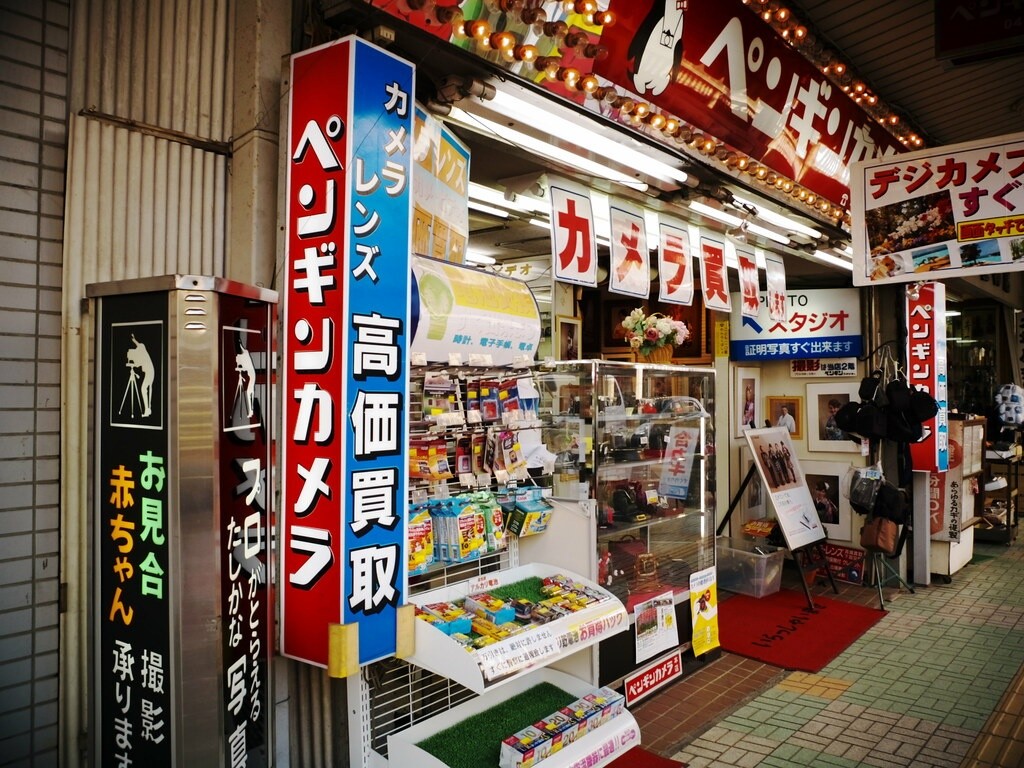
[618,488,637,504]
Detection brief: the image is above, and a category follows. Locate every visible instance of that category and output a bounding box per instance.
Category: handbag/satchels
[833,367,939,444]
[848,468,914,560]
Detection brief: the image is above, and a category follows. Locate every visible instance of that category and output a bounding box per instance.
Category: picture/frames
[805,381,864,453]
[798,459,853,542]
[733,366,761,438]
[765,395,803,440]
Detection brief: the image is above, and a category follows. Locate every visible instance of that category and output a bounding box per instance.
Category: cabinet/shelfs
[347,563,641,768]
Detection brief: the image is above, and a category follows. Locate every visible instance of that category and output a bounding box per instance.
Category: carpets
[717,590,890,674]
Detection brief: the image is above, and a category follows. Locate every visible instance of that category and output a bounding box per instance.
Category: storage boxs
[716,536,784,598]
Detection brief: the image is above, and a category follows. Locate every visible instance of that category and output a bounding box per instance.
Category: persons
[812,481,837,525]
[758,439,797,488]
[741,384,755,424]
[776,406,796,434]
[824,399,847,439]
